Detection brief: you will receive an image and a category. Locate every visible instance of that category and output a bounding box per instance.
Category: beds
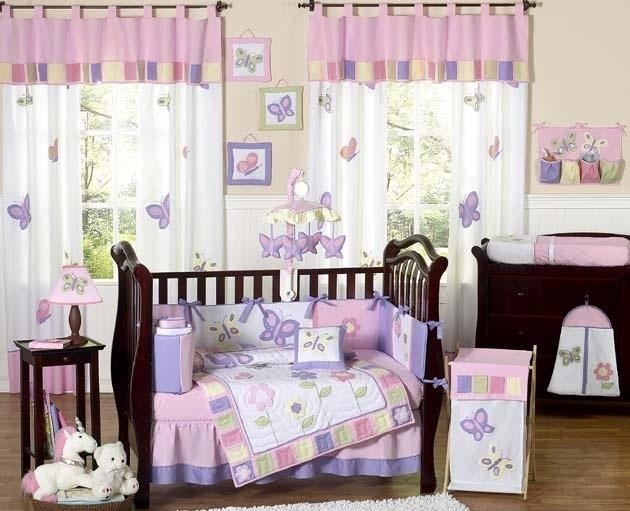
[103,234,454,511]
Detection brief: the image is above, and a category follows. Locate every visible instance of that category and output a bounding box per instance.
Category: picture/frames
[224,143,273,188]
[224,36,273,84]
[259,85,304,133]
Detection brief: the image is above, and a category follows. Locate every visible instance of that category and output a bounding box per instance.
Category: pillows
[293,325,347,373]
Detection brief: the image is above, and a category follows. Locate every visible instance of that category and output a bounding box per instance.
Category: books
[42,387,67,458]
[28,336,71,350]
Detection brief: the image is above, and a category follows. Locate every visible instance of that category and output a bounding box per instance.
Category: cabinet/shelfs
[12,338,108,483]
[471,229,630,416]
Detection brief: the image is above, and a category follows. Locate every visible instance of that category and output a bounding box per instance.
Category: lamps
[46,261,106,348]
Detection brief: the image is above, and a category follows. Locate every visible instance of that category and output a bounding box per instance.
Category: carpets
[175,487,472,511]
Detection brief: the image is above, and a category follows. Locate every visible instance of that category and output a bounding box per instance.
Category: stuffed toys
[15,410,97,504]
[90,440,139,500]
